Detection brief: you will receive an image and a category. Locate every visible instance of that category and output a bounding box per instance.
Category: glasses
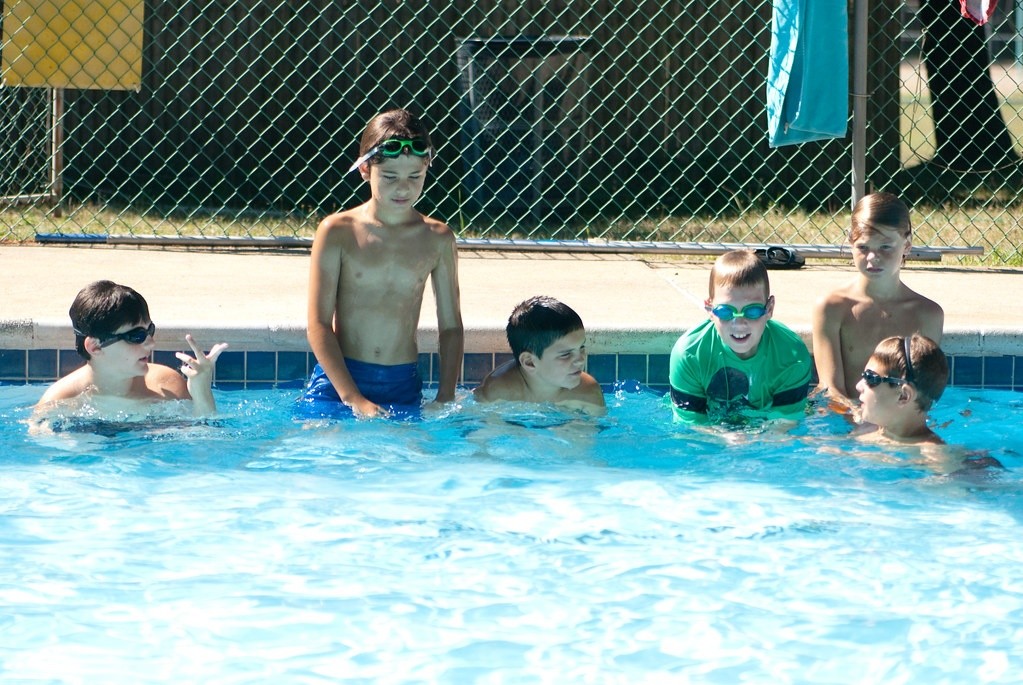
[105,322,155,345]
[375,138,431,157]
[861,369,904,389]
[710,303,767,321]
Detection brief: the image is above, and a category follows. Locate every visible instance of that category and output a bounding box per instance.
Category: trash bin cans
[456,37,586,223]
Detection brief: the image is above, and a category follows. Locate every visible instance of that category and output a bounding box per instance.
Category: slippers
[754,245,806,269]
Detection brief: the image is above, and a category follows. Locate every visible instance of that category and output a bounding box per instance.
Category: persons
[670,250,811,412]
[811,193,945,396]
[480,296,607,408]
[37,280,229,400]
[303,109,464,402]
[856,333,945,447]
